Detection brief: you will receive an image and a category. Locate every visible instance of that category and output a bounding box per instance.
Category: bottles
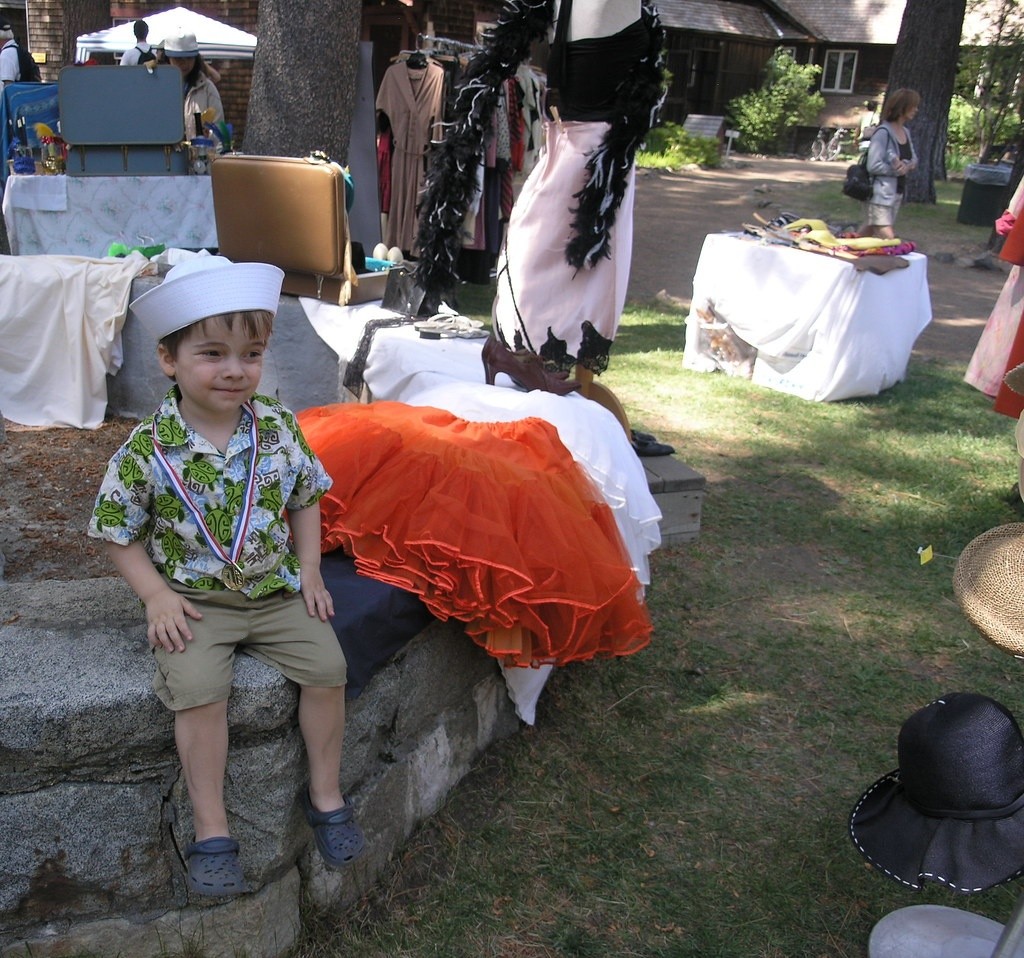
[183,107,235,174]
[887,149,902,177]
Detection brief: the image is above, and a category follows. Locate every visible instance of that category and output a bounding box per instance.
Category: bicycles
[797,121,829,162]
[819,123,854,161]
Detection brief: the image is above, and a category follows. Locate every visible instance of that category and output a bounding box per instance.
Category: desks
[0,173,218,256]
[681,231,934,402]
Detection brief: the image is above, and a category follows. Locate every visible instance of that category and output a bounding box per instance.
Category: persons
[156,32,222,142]
[492,0,669,378]
[0,18,22,96]
[121,19,157,68]
[87,250,370,900]
[856,88,920,240]
[84,52,100,65]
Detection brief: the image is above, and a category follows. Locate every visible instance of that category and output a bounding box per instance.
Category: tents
[75,6,259,63]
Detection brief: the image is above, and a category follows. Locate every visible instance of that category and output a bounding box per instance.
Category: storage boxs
[211,153,410,304]
[60,65,191,177]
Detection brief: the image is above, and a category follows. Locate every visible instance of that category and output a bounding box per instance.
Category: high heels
[482,335,581,396]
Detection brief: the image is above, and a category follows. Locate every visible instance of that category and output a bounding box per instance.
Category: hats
[151,39,165,48]
[164,32,199,58]
[0,25,14,38]
[129,249,285,340]
[848,692,1024,893]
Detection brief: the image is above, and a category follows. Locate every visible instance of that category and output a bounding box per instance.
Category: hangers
[406,33,479,70]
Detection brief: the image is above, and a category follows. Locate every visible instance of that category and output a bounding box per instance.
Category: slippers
[185,833,246,897]
[303,785,366,866]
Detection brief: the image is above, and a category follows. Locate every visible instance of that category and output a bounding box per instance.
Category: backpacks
[0,38,42,82]
[135,45,156,65]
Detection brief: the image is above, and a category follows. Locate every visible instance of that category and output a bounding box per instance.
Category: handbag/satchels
[842,164,873,201]
[861,126,876,140]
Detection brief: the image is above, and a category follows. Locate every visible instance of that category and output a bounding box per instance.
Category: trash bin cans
[957,164,1011,228]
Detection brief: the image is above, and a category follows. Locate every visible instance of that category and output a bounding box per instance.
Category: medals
[221,562,245,591]
